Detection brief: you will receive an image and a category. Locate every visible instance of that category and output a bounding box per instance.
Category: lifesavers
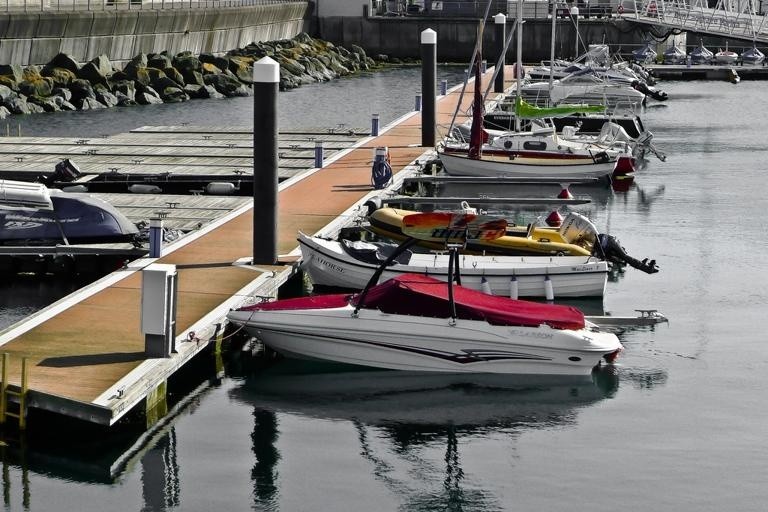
[617,6,624,13]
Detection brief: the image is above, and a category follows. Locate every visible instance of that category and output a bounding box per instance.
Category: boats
[662,38,765,66]
